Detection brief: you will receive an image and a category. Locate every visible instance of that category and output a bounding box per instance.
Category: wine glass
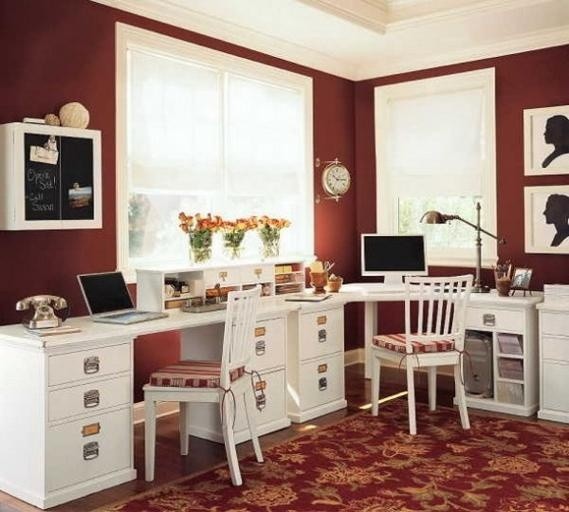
[310,271,328,294]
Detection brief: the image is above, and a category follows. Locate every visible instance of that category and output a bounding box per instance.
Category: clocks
[312,154,353,206]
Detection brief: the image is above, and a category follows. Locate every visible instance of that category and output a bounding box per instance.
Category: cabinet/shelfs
[275,289,353,425]
[0,120,106,234]
[531,292,568,427]
[133,254,309,316]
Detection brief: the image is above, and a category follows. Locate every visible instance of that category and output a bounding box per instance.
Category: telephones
[16,295,68,329]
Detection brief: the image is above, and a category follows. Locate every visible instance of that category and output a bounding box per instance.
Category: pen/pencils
[494,260,512,280]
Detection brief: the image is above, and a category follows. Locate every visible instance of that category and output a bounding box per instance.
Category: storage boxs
[494,331,526,407]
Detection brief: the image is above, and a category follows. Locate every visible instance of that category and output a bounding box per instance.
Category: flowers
[214,213,261,261]
[253,214,292,257]
[176,208,224,261]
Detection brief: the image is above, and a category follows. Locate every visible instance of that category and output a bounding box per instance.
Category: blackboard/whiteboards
[13,123,103,230]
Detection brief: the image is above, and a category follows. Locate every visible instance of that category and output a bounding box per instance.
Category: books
[285,293,332,301]
[276,264,303,294]
[495,333,523,406]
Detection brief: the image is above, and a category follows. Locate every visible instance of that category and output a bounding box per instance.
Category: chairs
[137,283,269,489]
[367,269,479,436]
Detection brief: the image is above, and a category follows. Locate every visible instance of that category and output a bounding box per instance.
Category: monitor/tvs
[361,233,429,293]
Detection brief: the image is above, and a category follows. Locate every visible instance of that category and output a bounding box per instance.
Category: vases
[221,237,248,261]
[257,232,281,258]
[183,228,214,264]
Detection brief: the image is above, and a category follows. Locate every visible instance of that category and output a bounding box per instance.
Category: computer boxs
[464,335,492,398]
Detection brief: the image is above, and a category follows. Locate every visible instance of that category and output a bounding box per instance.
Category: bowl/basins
[327,278,344,293]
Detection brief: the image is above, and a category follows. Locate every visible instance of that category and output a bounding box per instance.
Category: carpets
[73,385,568,511]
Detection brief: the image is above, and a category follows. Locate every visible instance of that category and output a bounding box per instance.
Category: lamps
[416,199,508,295]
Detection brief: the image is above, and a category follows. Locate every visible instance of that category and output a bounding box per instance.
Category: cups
[493,268,515,297]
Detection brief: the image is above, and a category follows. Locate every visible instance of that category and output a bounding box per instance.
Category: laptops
[76,271,169,324]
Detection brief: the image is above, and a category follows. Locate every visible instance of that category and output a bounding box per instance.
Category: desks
[0,281,302,511]
[327,281,545,420]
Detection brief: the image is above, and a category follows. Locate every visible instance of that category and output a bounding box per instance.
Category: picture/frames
[507,264,535,291]
[520,102,569,178]
[521,183,569,256]
